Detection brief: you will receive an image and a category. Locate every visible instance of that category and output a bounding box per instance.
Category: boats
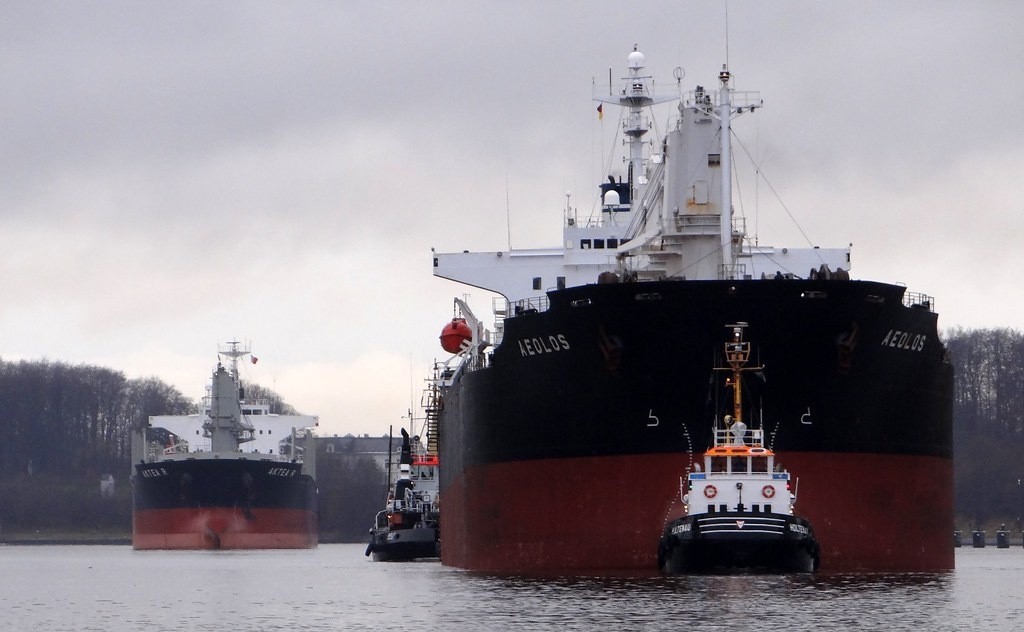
[364,426,440,563]
[131,334,319,549]
[656,322,822,575]
[423,0,957,578]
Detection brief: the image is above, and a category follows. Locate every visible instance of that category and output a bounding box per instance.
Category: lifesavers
[704,484,717,498]
[762,485,776,499]
